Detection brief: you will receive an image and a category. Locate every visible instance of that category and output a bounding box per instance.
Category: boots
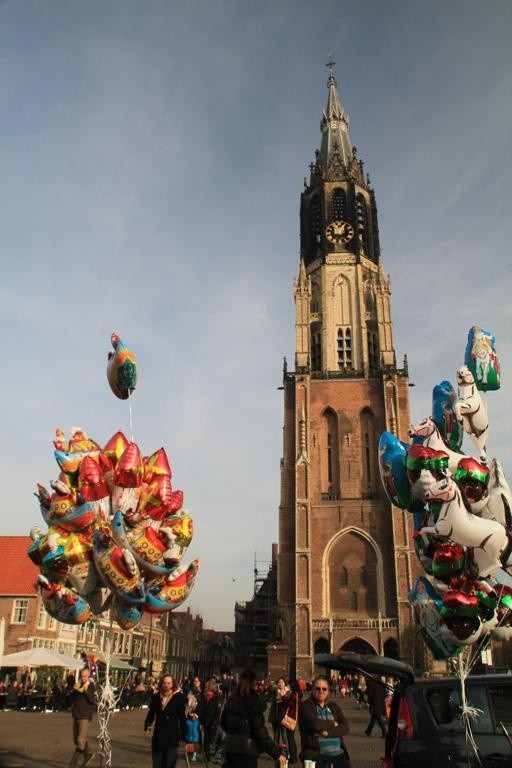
[191,752,197,762]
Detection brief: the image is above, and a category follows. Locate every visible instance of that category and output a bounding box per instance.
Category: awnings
[88,652,134,669]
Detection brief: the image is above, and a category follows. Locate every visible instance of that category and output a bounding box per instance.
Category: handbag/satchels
[280,714,297,732]
[208,726,227,765]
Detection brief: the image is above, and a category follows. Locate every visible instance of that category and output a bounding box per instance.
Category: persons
[143,674,186,767]
[174,673,234,765]
[258,672,370,709]
[268,677,303,763]
[1,669,159,712]
[70,669,94,766]
[363,677,398,737]
[298,677,351,767]
[221,669,288,766]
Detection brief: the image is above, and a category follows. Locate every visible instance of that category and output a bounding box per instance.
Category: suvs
[313,650,511,768]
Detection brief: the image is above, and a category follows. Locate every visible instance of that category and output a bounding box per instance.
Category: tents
[1,647,86,669]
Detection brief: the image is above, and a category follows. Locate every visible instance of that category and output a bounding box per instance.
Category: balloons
[107,334,137,400]
[376,418,511,663]
[465,326,501,390]
[28,427,200,637]
[432,365,489,459]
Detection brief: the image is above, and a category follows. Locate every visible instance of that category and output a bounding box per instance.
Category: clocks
[325,219,355,246]
[0,688,59,714]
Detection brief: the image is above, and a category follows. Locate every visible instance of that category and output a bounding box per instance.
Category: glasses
[314,687,327,691]
